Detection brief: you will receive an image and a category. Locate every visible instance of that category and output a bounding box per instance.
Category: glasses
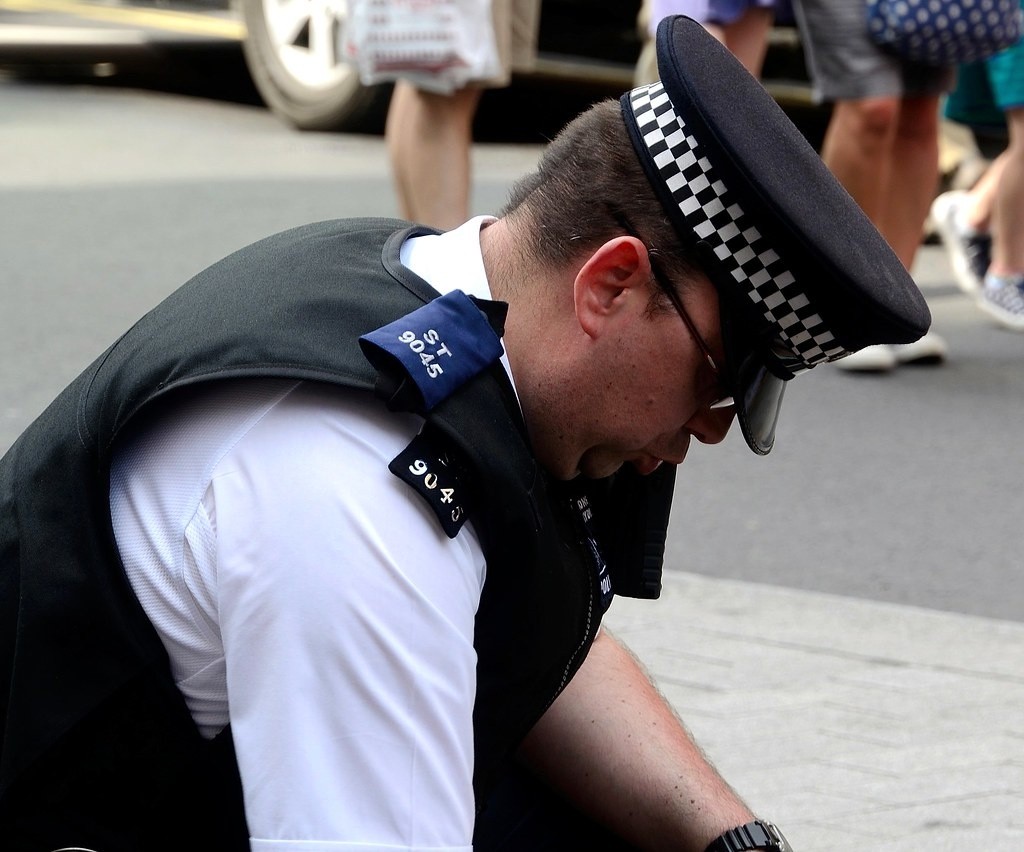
[592,197,735,412]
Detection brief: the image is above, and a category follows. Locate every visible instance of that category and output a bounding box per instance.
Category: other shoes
[840,346,896,372]
[895,333,948,364]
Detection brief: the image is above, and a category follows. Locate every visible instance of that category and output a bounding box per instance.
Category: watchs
[701,819,793,852]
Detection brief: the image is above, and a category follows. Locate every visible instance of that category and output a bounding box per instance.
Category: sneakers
[976,274,1024,332]
[930,191,995,288]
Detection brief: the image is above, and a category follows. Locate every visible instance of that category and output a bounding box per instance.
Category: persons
[929,0,1024,328]
[384,1,541,233]
[635,1,774,90]
[0,15,931,852]
[792,0,956,373]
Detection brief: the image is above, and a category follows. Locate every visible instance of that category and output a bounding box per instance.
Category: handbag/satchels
[343,0,499,96]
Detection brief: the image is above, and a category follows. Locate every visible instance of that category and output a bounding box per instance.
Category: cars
[232,0,990,238]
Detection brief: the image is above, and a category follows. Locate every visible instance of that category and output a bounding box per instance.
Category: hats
[621,16,931,456]
